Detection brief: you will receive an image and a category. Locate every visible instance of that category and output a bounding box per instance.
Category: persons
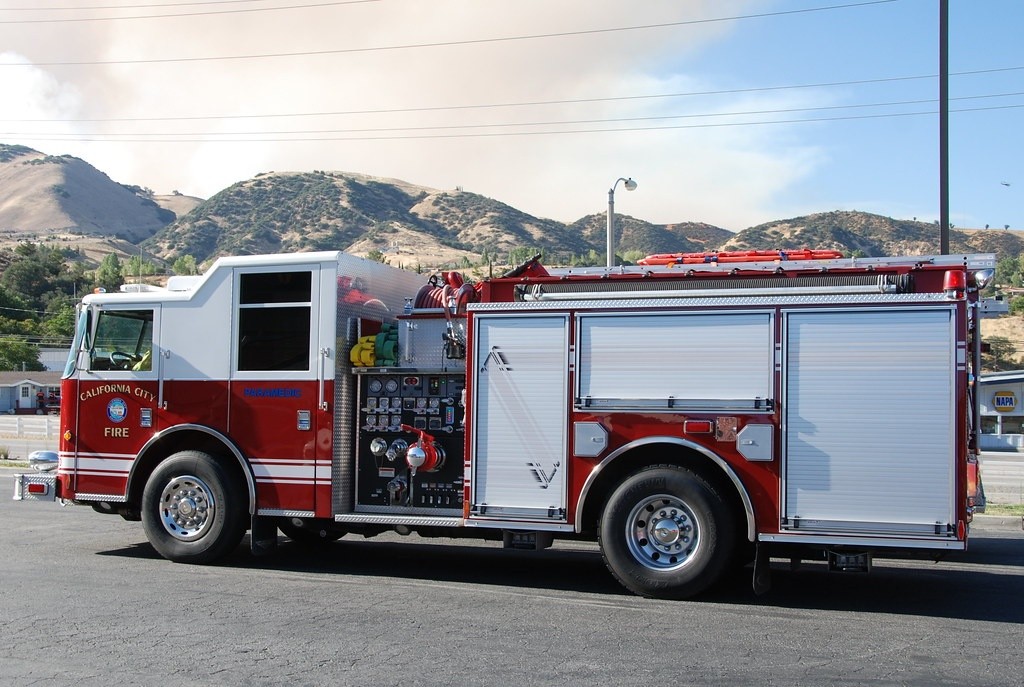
[36,389,45,407]
[130,350,152,371]
[49,389,55,403]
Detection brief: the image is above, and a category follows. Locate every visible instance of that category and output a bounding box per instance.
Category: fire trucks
[14,249,1009,602]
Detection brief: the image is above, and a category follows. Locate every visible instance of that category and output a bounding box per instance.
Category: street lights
[605,177,637,267]
